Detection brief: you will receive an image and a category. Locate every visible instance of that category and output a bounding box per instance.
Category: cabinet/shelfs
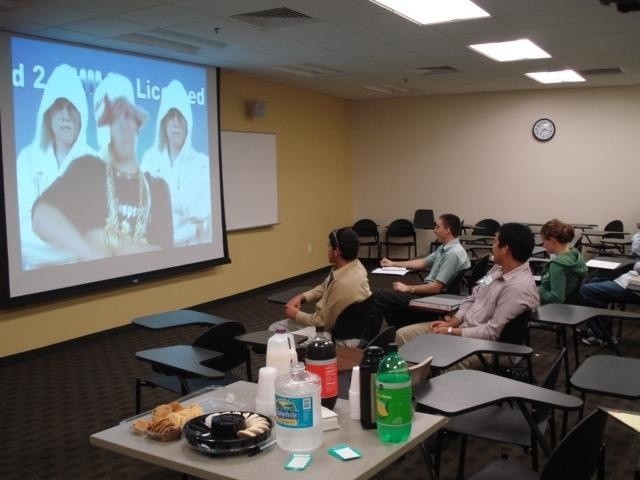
[374,341,413,445]
[265,327,298,375]
[272,361,324,451]
[304,326,339,410]
[360,346,385,429]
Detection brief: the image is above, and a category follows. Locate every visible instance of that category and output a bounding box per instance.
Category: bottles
[92,73,136,146]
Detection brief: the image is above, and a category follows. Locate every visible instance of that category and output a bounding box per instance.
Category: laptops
[205,411,270,439]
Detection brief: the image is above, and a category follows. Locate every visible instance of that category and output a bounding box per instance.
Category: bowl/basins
[104,140,153,255]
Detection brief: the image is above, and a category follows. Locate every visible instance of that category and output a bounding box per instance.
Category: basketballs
[133,402,202,441]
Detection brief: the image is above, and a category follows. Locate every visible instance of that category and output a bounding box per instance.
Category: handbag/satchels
[448,326,453,335]
[410,285,415,293]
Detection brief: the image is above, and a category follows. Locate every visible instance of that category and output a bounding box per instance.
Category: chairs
[576,328,617,347]
[130,208,640,479]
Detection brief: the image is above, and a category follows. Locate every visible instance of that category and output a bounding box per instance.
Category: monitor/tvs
[382,265,407,271]
[409,293,467,311]
[629,275,640,284]
[626,282,640,292]
[321,405,340,432]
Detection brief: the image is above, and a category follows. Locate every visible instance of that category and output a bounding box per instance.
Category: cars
[349,365,359,420]
[254,367,279,418]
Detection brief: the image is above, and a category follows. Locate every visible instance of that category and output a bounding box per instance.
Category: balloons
[532,118,556,141]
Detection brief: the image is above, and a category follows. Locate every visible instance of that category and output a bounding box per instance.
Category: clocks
[532,118,556,141]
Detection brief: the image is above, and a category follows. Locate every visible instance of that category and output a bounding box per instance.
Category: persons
[395,222,541,373]
[139,79,212,246]
[577,229,640,345]
[31,71,177,261]
[268,229,373,352]
[373,214,471,327]
[13,63,104,273]
[531,218,588,326]
